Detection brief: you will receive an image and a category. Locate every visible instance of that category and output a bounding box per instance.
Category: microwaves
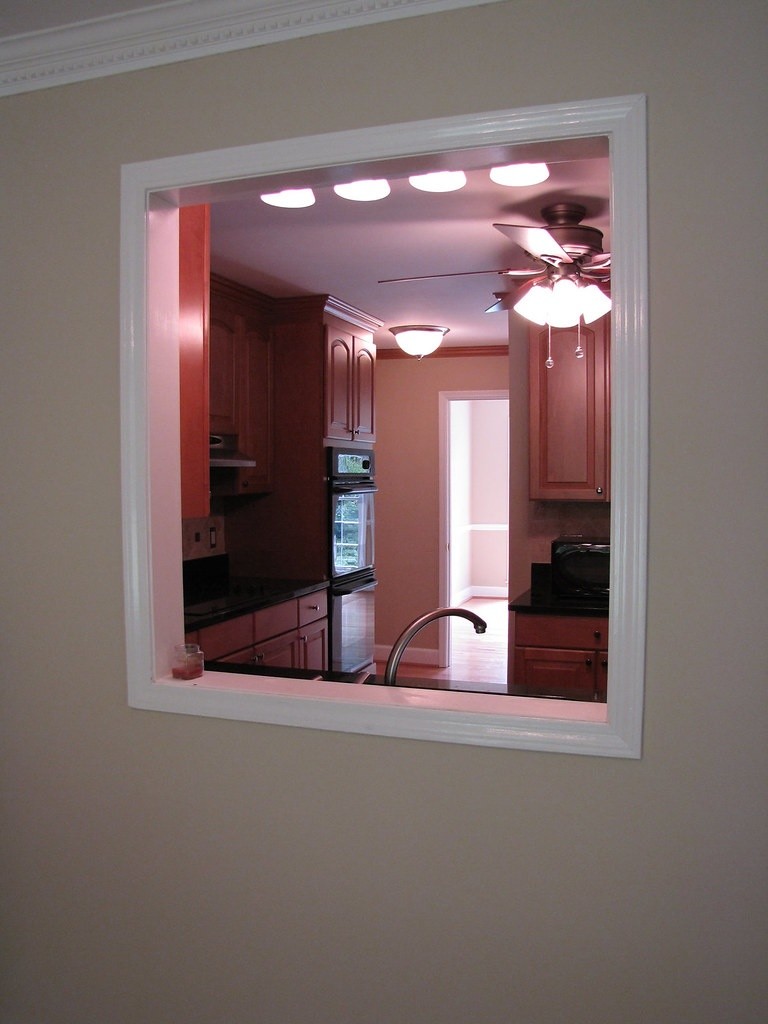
[550,534,610,602]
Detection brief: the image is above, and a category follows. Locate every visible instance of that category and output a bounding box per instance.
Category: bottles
[171,644,203,680]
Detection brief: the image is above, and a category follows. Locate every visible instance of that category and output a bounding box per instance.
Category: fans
[376,204,612,314]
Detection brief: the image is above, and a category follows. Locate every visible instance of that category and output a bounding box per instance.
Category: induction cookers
[183,553,330,633]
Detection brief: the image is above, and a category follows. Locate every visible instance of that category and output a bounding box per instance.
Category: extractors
[209,433,281,503]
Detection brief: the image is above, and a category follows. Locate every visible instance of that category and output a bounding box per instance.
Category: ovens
[325,446,378,672]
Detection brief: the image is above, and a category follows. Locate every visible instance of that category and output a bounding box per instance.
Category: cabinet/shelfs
[516,612,609,702]
[494,292,613,503]
[185,589,330,672]
[208,272,387,494]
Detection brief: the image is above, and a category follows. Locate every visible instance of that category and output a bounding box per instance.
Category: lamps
[390,324,450,360]
[514,276,612,368]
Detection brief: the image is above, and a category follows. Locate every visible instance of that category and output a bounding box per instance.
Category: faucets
[384,607,487,687]
